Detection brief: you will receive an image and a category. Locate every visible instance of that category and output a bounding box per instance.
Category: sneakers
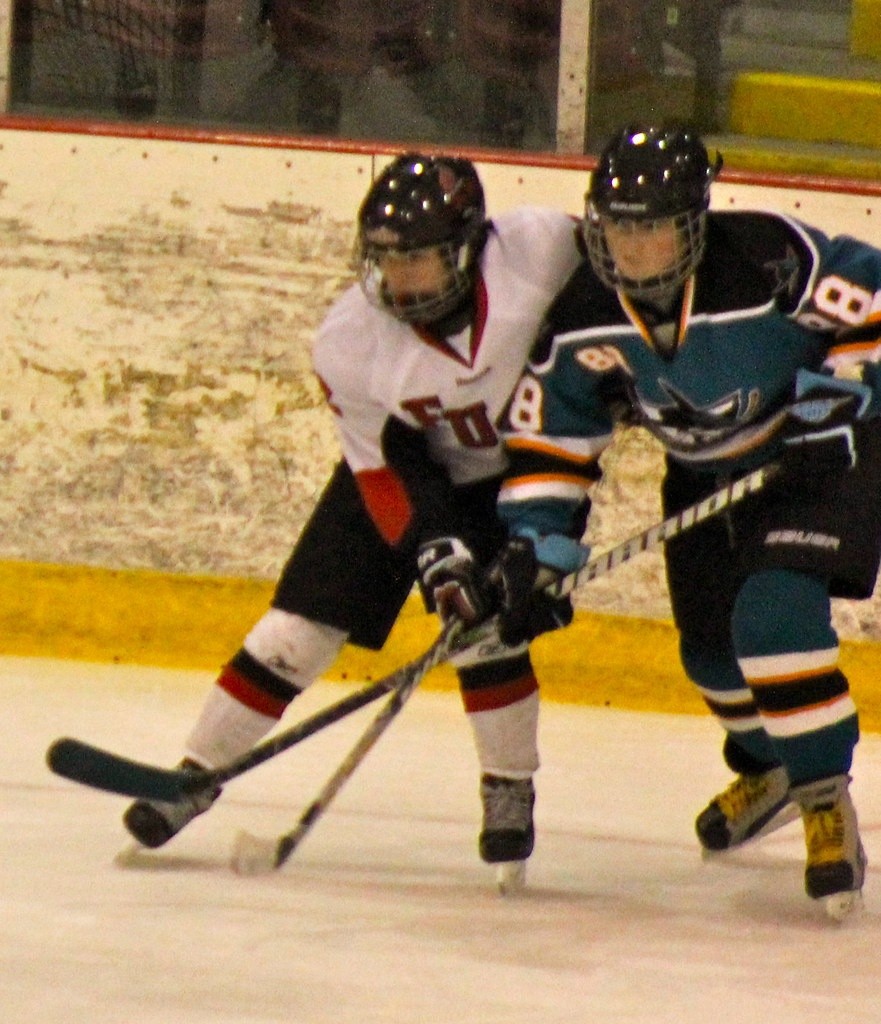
[696,767,803,863]
[476,773,539,890]
[123,759,223,849]
[789,776,869,923]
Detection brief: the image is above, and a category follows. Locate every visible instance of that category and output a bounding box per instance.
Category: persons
[124,151,589,895]
[493,117,881,923]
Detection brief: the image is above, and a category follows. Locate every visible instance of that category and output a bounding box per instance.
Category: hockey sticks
[227,554,474,877]
[44,458,782,804]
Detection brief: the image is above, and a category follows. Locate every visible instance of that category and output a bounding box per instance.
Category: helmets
[357,151,485,331]
[582,120,708,297]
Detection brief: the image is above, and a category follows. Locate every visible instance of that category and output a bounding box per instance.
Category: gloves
[499,531,591,649]
[415,539,485,633]
[488,539,539,611]
[779,369,872,501]
